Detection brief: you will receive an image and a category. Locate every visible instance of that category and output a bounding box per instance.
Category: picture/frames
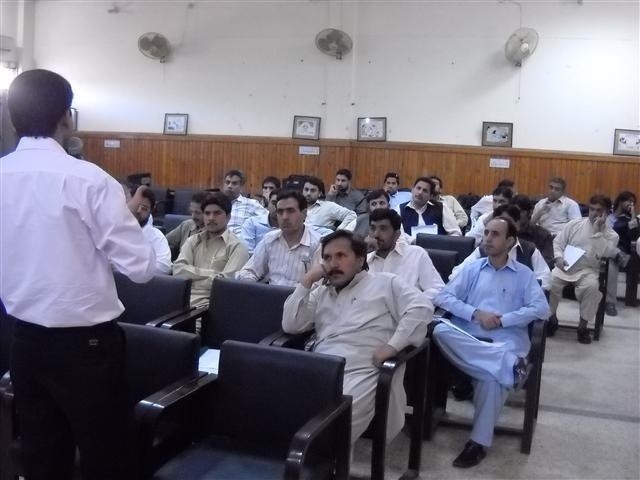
[357,116,387,142]
[160,112,188,135]
[612,129,640,156]
[481,120,512,146]
[292,115,322,140]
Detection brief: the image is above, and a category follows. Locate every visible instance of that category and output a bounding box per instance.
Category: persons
[434,216,552,468]
[604,191,640,316]
[0,68,158,480]
[466,178,619,344]
[133,169,468,468]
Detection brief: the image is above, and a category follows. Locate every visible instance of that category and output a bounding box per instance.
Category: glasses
[69,106,78,134]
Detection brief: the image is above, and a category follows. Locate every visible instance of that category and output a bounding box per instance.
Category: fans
[505,26,540,68]
[315,27,354,61]
[137,31,170,65]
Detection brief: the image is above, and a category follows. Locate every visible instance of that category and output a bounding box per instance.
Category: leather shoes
[453,439,488,467]
[513,356,534,393]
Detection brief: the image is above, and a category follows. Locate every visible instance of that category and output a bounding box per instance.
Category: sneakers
[605,303,617,316]
[547,315,558,336]
[577,325,592,344]
[625,299,640,306]
